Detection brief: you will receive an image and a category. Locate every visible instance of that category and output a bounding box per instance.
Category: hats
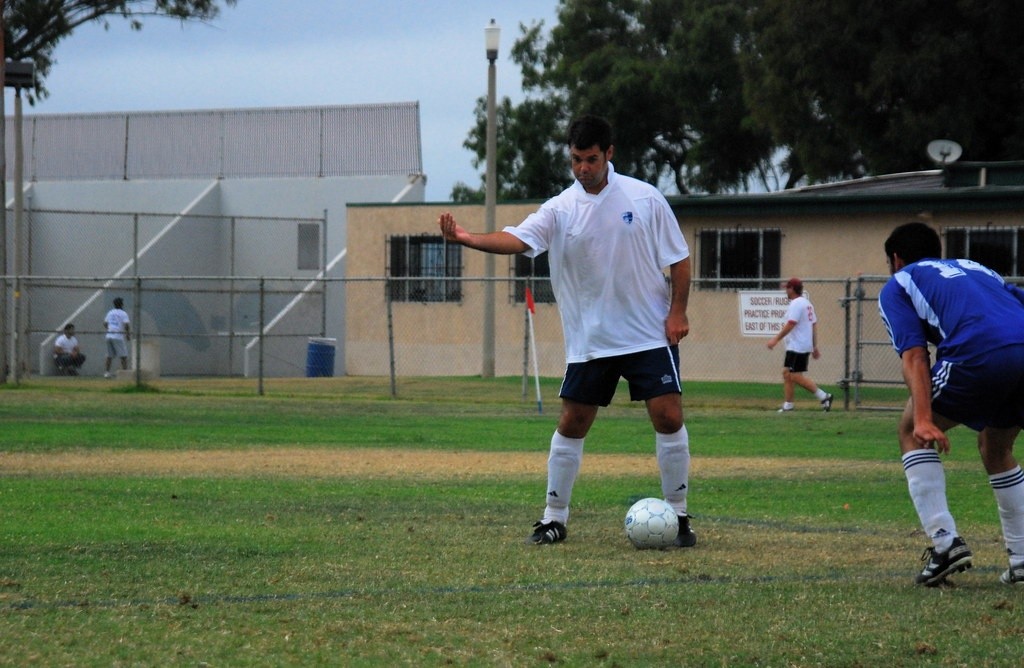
[780,278,804,288]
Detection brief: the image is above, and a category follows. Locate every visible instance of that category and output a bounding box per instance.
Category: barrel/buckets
[131,339,161,380]
[306,338,336,377]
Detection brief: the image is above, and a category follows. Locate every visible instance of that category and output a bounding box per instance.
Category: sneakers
[527,520,567,544]
[821,393,834,411]
[915,537,974,586]
[672,514,696,546]
[778,408,793,412]
[999,564,1024,587]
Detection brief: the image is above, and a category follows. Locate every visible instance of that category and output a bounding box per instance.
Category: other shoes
[67,369,78,375]
[105,371,109,377]
[57,365,63,375]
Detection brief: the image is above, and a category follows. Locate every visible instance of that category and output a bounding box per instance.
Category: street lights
[481,16,500,378]
[4,62,36,384]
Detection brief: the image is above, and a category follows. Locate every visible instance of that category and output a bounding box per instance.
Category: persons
[876,222,1024,586]
[437,115,699,548]
[766,278,833,413]
[103,297,132,378]
[52,324,87,376]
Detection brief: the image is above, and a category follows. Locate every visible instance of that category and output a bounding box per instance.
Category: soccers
[623,496,681,553]
